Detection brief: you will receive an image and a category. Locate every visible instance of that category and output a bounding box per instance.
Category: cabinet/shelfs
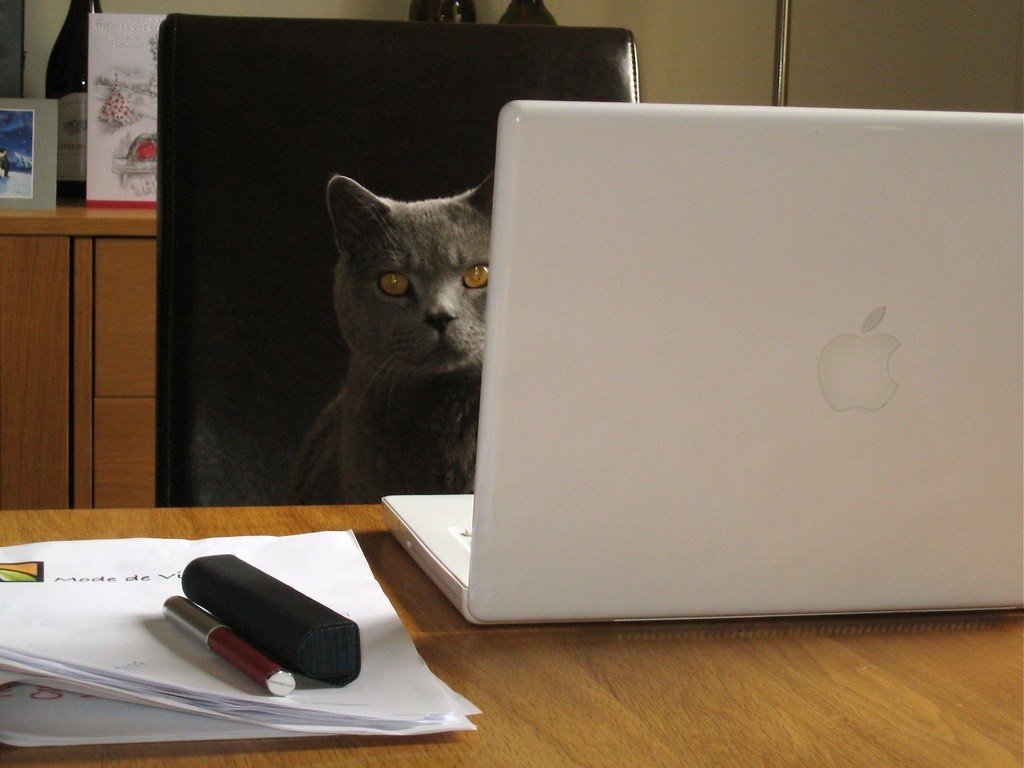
[0,236,158,511]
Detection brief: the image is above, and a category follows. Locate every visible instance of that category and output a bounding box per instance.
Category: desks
[0,498,1021,768]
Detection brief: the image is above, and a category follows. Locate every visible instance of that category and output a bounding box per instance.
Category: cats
[284,172,494,505]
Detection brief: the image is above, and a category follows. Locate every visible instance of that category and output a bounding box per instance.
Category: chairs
[151,13,644,508]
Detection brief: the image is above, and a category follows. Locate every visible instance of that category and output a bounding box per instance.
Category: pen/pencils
[163,597,295,697]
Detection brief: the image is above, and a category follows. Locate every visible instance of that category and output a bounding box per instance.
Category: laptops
[384,100,1024,625]
